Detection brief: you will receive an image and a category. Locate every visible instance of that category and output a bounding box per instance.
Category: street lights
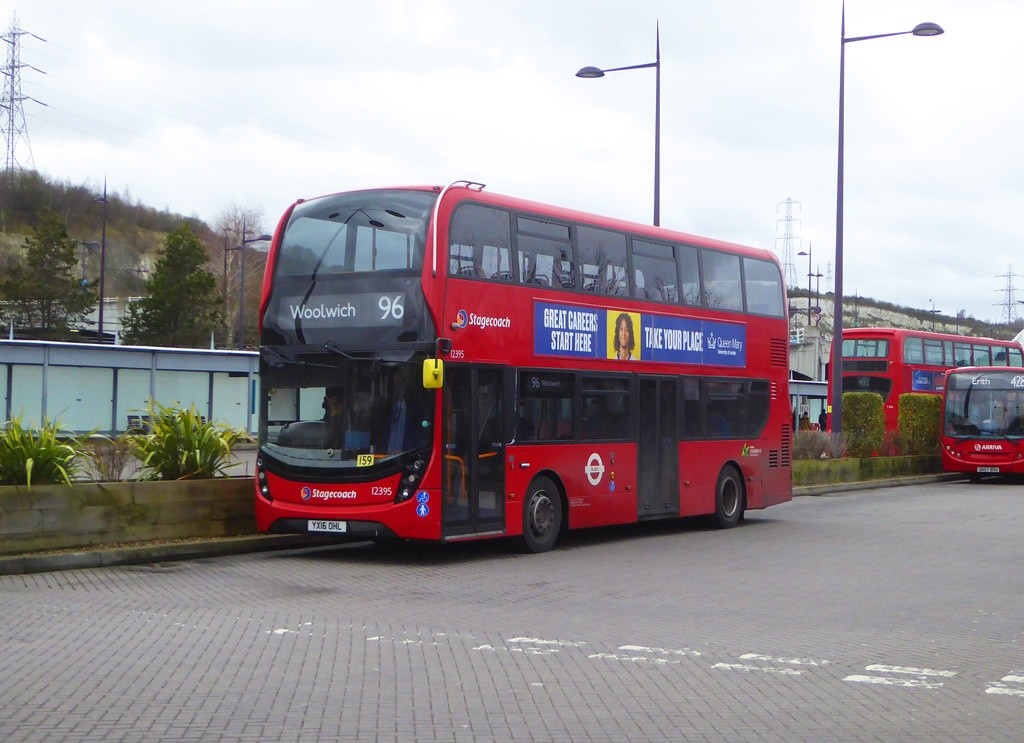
[927,296,939,332]
[832,0,945,457]
[798,242,815,327]
[815,265,823,327]
[80,169,106,343]
[223,216,272,349]
[574,17,661,226]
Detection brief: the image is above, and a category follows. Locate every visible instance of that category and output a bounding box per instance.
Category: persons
[613,313,636,360]
[322,386,356,432]
[792,407,811,431]
[819,409,827,433]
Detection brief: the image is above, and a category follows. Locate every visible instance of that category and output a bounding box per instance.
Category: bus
[939,366,1024,482]
[255,179,793,554]
[825,327,1024,458]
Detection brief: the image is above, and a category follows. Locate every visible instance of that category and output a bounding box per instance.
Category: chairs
[491,271,513,280]
[585,282,607,293]
[456,265,486,278]
[911,355,921,363]
[525,275,549,286]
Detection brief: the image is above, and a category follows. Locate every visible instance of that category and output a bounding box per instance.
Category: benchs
[127,415,206,432]
